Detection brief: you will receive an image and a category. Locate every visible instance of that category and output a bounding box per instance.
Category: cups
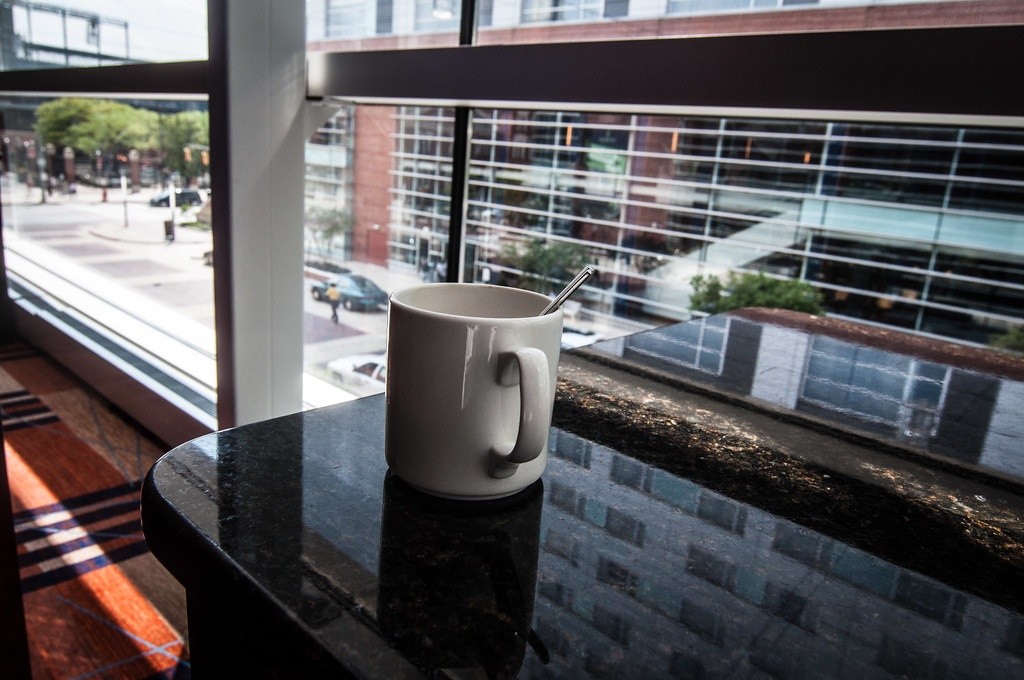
[383,282,564,500]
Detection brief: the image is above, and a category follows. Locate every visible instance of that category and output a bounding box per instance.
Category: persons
[326,282,341,324]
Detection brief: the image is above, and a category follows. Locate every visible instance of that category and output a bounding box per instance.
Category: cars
[311,273,389,314]
[149,189,202,209]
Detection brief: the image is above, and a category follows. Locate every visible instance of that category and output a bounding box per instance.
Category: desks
[142,392,1024,680]
[567,306,1023,499]
